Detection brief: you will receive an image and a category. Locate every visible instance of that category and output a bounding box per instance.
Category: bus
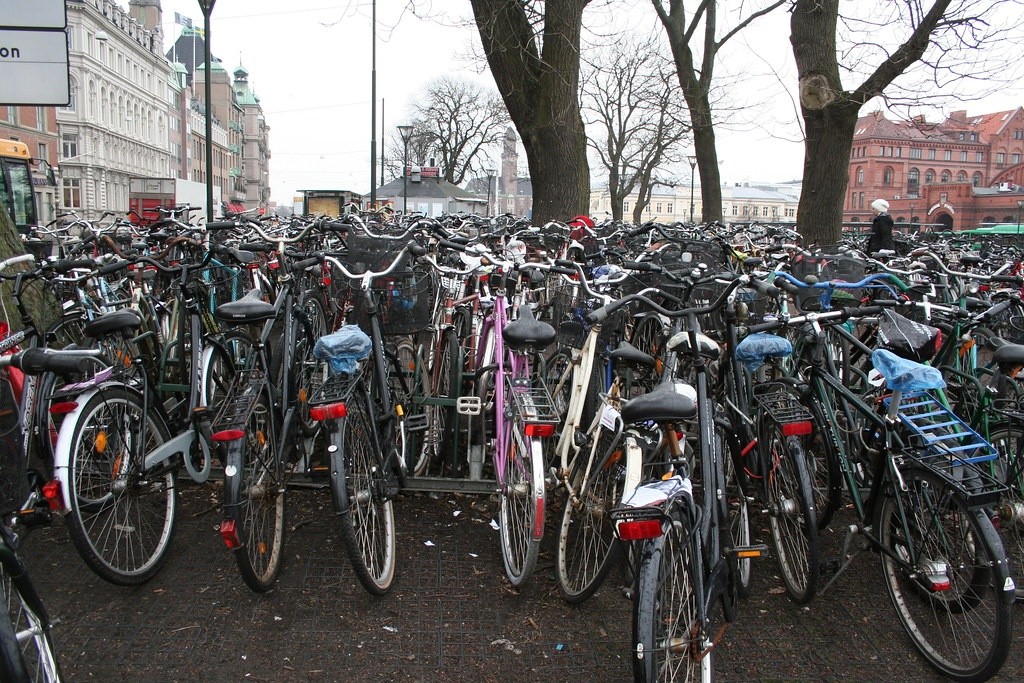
[0,139,58,236]
[933,224,1024,250]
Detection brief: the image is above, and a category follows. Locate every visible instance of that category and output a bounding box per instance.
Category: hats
[872,199,889,213]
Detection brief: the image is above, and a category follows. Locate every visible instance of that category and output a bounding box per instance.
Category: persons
[866,198,895,260]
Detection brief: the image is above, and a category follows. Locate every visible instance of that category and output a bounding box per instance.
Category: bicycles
[0,199,1023,682]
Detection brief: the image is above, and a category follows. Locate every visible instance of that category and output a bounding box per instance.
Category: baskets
[347,221,418,275]
[350,272,430,335]
[956,251,981,262]
[790,245,866,311]
[553,283,624,354]
[660,239,731,336]
[171,265,243,316]
[622,272,684,313]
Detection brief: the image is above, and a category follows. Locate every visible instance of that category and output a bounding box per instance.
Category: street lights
[909,201,915,233]
[1017,200,1023,241]
[484,169,494,214]
[398,123,414,216]
[688,156,698,221]
